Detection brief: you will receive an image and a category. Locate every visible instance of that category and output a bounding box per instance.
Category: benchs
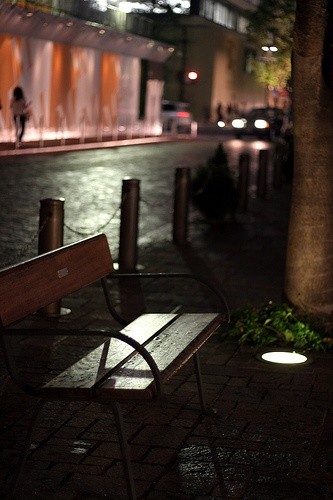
[0,232,232,500]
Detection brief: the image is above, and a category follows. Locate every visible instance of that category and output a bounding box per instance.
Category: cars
[158,99,194,129]
[234,107,286,138]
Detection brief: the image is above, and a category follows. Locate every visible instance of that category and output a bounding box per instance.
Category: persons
[9,86,33,149]
[216,100,293,129]
